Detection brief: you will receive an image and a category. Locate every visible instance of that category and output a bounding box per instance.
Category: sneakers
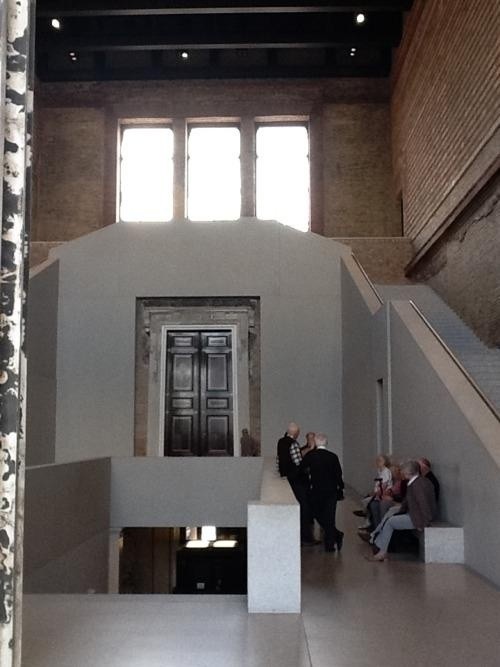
[352,511,385,561]
[302,531,343,553]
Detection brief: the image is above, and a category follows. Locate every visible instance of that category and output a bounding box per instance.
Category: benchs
[411,518,466,564]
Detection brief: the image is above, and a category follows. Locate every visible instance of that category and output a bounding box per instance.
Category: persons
[272,422,345,552]
[351,452,441,561]
